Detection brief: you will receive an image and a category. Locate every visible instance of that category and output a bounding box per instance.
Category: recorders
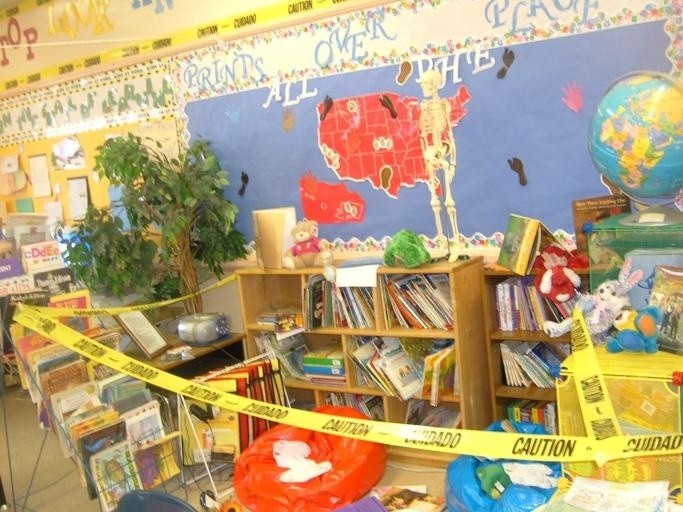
[178,311,232,345]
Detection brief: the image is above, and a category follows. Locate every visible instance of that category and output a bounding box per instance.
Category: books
[177,356,291,466]
[334,485,448,512]
[496,213,575,276]
[496,275,582,435]
[253,273,461,428]
[252,206,296,269]
[2,213,184,512]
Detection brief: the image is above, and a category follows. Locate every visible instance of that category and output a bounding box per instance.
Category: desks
[125,330,247,391]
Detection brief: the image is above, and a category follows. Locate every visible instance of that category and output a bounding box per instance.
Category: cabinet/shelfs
[479,262,621,471]
[236,258,493,471]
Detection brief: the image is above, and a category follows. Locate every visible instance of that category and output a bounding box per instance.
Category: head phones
[181,396,219,423]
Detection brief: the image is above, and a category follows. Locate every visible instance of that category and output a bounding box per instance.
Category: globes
[590,68,683,224]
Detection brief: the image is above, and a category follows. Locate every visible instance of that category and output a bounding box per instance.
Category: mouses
[201,490,220,512]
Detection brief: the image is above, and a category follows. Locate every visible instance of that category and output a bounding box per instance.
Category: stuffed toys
[282,220,332,270]
[541,255,644,350]
